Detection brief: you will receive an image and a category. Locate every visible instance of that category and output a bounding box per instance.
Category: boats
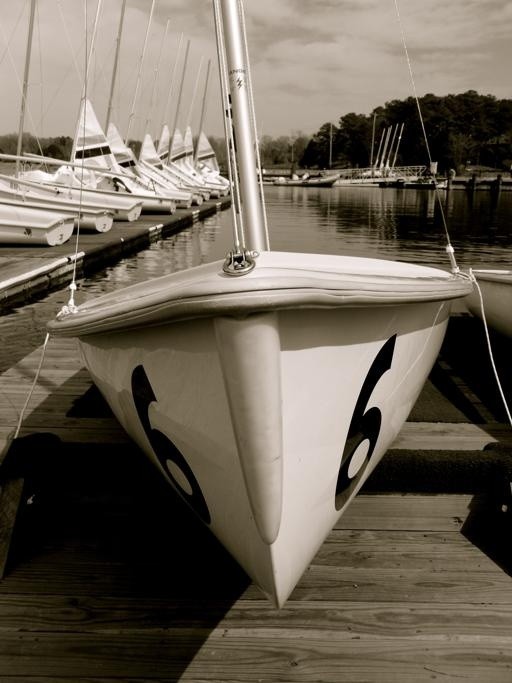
[461,268,511,338]
[271,174,340,187]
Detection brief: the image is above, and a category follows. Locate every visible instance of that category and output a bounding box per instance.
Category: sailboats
[335,124,424,185]
[42,1,474,612]
